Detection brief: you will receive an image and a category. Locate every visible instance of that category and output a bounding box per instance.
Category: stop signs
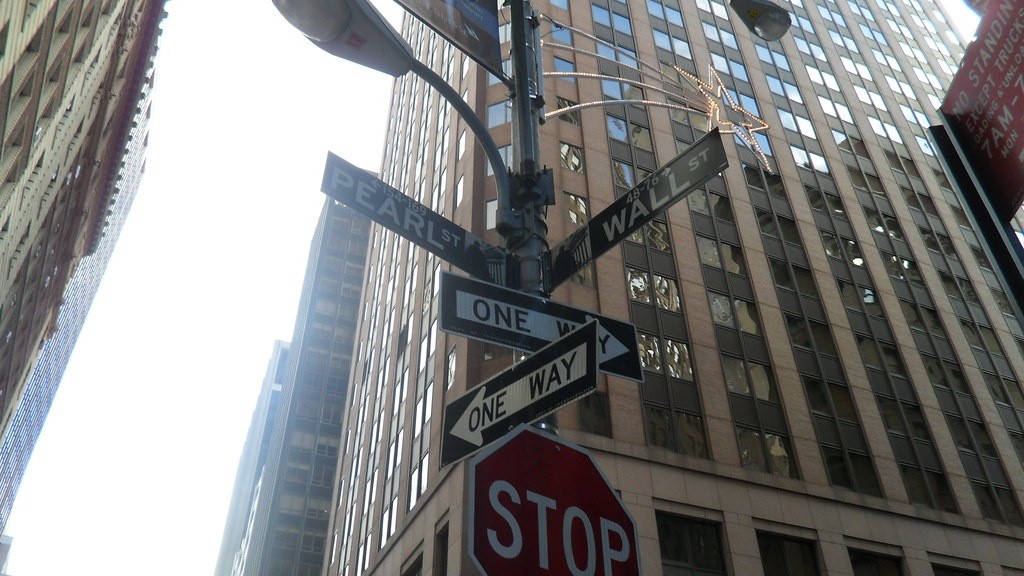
[462,423,642,576]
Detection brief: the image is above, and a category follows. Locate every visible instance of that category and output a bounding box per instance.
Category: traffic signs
[320,150,508,287]
[548,125,730,291]
